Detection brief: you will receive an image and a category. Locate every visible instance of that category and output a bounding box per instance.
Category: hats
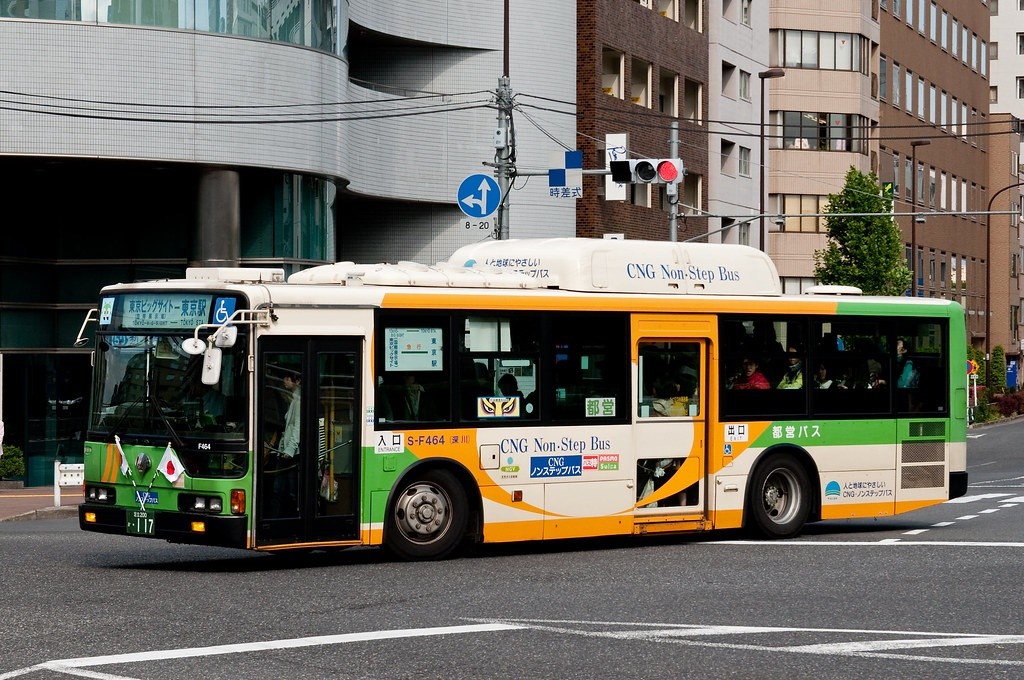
[742,353,757,362]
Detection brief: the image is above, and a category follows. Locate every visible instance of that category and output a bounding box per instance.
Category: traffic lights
[610,158,684,184]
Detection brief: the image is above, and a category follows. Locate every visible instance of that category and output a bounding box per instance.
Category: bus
[76,239,970,562]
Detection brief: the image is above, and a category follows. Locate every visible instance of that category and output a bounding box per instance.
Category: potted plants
[0,445,25,489]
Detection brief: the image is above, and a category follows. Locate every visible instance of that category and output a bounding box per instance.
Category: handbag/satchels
[320,470,338,503]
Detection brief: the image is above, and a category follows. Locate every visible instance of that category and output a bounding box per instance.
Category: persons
[276,370,301,518]
[726,338,920,390]
[638,373,698,506]
[392,362,527,421]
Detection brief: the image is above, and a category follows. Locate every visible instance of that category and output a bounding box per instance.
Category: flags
[115,438,129,475]
[157,445,185,483]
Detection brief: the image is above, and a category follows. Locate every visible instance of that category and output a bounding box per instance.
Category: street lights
[909,139,931,297]
[758,67,788,252]
